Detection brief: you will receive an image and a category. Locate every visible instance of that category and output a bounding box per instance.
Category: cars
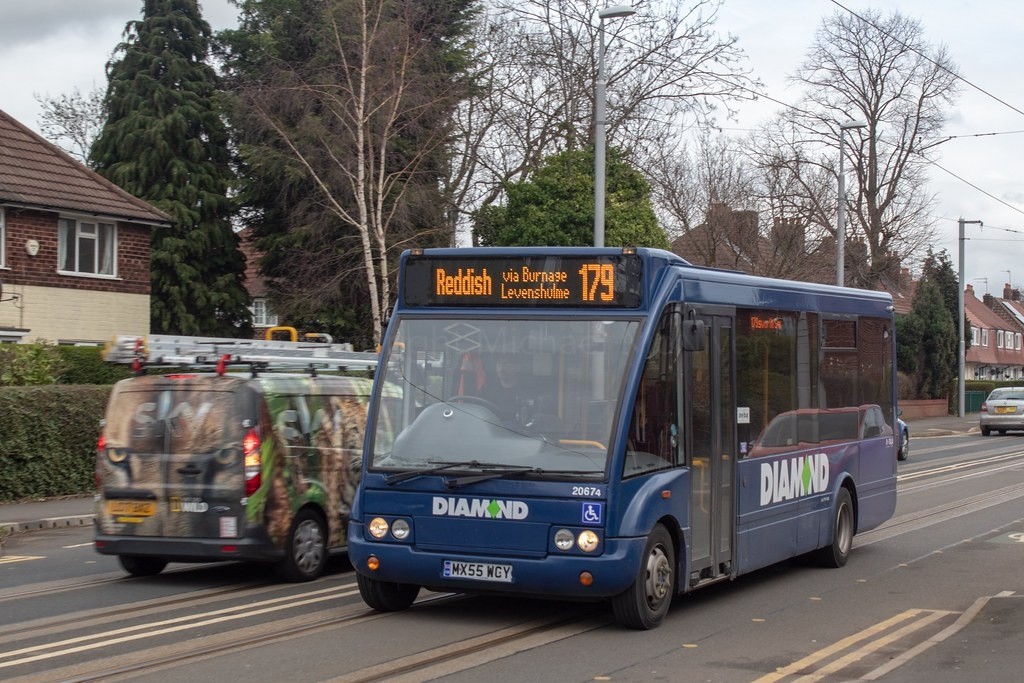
[897,405,910,461]
[979,386,1024,436]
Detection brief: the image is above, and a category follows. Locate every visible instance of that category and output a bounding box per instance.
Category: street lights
[593,6,639,401]
[835,118,870,287]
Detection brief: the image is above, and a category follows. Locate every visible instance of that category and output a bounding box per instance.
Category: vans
[90,360,425,583]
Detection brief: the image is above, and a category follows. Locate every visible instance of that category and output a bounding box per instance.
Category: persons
[478,352,533,421]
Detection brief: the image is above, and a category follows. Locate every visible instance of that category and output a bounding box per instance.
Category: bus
[346,245,898,633]
